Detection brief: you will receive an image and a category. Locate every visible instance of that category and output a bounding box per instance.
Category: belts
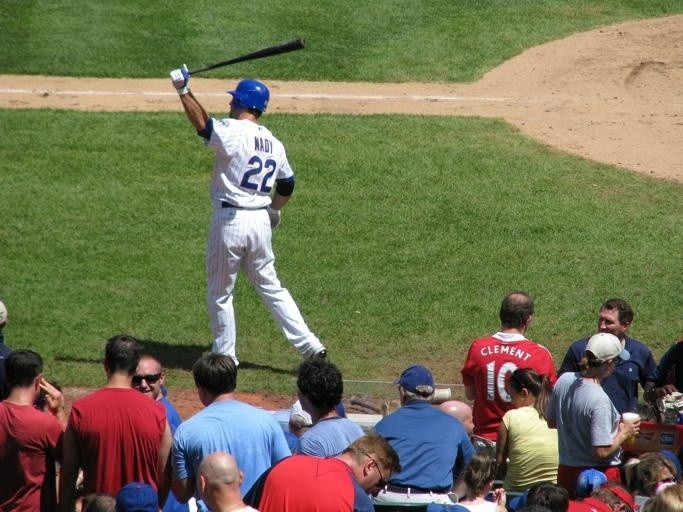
[384,483,451,495]
[221,201,268,212]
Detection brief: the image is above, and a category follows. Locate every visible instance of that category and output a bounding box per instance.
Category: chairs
[622,422,683,453]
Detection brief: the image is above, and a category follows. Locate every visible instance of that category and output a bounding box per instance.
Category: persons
[170,62,330,365]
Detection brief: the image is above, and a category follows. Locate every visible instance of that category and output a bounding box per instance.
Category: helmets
[226,78,270,115]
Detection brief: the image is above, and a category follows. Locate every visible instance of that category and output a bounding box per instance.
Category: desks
[265,410,383,434]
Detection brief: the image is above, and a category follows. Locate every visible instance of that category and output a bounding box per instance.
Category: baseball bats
[188,39,305,75]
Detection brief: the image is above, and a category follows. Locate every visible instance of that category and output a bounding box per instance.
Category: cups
[623,411,639,443]
[433,387,451,401]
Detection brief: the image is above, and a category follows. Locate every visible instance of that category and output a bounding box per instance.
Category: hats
[659,450,682,482]
[585,332,631,368]
[393,364,436,398]
[113,482,160,512]
[574,465,636,511]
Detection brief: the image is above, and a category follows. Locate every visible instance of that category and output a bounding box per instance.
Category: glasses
[132,373,162,387]
[363,450,386,490]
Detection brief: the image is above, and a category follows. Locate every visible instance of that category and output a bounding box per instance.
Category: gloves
[168,63,191,95]
[267,203,282,229]
